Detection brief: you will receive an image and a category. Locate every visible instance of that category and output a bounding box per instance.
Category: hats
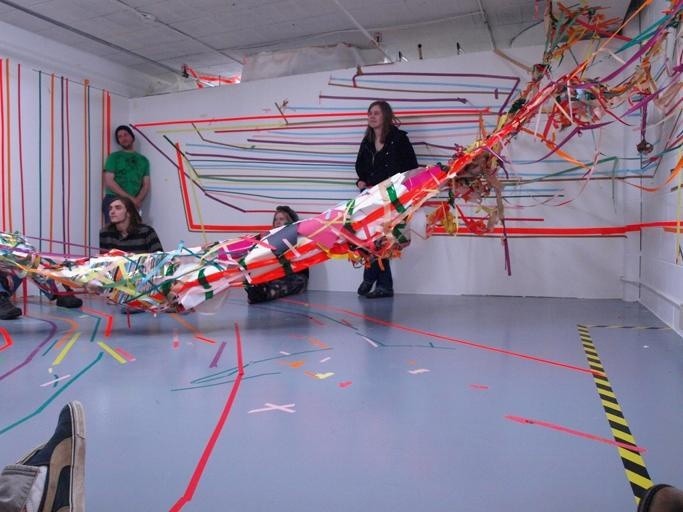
[116,126,134,144]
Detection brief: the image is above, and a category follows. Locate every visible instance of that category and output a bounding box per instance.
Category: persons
[99,196,162,255]
[102,125,150,228]
[0,257,82,322]
[0,399,86,511]
[241,205,308,303]
[351,100,418,299]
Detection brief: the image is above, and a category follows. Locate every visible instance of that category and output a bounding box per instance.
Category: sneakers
[56,295,83,308]
[23,399,87,512]
[357,281,373,295]
[0,292,22,319]
[366,289,394,299]
